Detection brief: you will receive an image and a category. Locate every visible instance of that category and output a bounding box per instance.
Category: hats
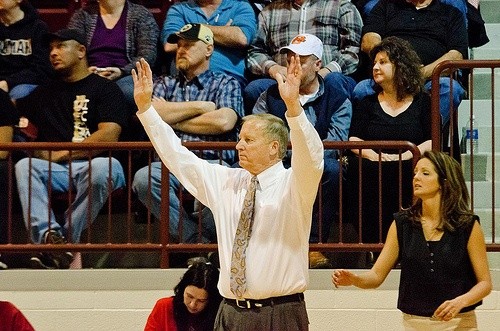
[166,23,214,45]
[279,33,323,60]
[40,29,87,50]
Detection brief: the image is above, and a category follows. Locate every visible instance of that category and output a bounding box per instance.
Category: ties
[229,176,258,299]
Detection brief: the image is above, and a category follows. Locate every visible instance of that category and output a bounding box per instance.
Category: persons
[144,261,224,331]
[0,0,489,271]
[132,52,324,331]
[331,150,492,331]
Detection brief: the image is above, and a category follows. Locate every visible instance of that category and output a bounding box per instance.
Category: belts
[225,293,304,308]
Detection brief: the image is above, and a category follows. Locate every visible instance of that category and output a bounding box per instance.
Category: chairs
[35,7,162,214]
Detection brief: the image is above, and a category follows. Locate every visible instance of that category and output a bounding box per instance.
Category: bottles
[466,116,479,154]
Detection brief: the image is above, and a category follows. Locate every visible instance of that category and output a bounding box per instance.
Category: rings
[294,72,297,77]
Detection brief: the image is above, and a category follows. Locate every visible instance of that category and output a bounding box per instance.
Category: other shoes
[188,243,220,269]
[310,251,329,269]
[43,229,74,265]
[29,253,56,269]
